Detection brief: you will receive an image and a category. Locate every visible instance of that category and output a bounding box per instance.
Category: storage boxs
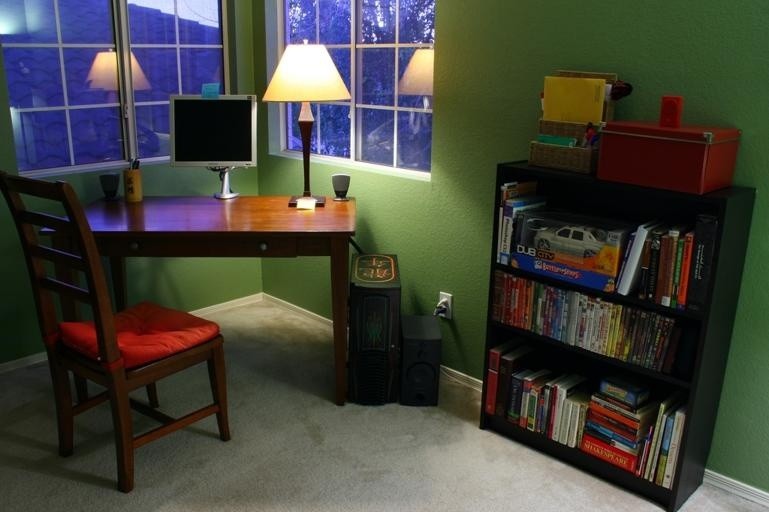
[593,118,744,197]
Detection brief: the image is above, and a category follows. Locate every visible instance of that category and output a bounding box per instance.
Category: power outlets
[436,291,454,321]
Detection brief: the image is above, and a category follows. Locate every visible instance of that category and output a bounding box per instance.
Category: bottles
[585,122,596,141]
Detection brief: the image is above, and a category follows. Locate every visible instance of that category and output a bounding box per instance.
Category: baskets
[528,71,617,173]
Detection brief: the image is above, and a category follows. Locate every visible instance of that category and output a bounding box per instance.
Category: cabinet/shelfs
[476,160,758,511]
[528,68,620,177]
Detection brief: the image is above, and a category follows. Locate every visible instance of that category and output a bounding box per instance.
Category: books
[497,181,546,269]
[614,221,716,313]
[485,345,686,490]
[491,270,676,371]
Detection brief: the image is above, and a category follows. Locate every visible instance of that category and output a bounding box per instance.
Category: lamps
[85,46,151,105]
[394,49,434,98]
[260,38,354,209]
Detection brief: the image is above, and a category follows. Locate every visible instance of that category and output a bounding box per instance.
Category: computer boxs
[349,254,401,407]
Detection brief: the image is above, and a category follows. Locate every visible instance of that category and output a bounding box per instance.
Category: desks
[45,191,356,408]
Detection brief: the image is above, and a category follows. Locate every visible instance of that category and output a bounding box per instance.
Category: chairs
[0,170,234,495]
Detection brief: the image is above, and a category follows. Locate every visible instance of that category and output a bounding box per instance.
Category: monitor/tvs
[169,94,259,199]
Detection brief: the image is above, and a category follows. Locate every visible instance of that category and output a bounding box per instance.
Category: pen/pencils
[130,158,140,169]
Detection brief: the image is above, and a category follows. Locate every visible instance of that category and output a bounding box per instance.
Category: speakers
[400,316,442,407]
[99,173,121,201]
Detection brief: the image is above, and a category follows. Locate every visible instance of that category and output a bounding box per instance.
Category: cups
[659,95,683,125]
[100,173,119,200]
